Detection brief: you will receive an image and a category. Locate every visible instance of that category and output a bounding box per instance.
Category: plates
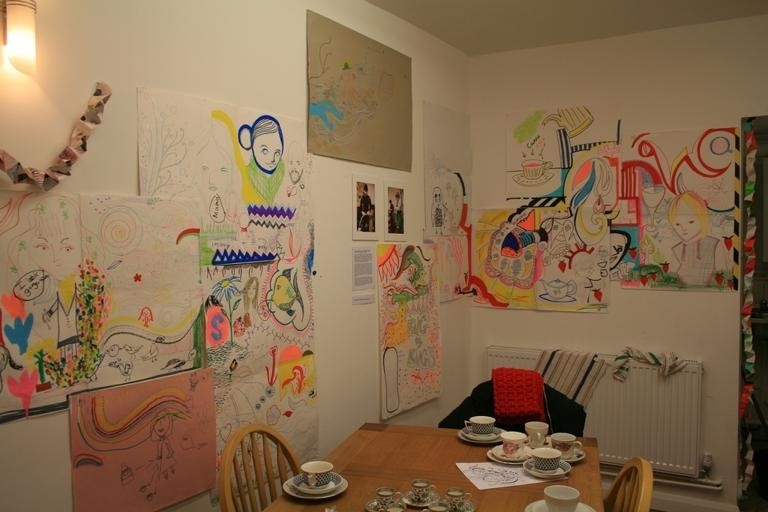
[282,475,348,500]
[365,494,479,512]
[457,427,597,512]
[512,173,554,186]
[539,293,575,303]
[290,471,343,494]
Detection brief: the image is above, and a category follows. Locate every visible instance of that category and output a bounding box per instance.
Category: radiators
[486,345,707,478]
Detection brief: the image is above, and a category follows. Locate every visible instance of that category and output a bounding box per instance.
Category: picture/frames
[383,181,410,240]
[353,177,383,239]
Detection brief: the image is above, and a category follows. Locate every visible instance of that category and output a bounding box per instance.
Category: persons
[356,184,375,231]
[388,191,404,233]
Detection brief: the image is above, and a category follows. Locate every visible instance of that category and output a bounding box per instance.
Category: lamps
[5,2,37,80]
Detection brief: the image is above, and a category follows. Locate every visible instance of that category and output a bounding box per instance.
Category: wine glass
[642,186,665,232]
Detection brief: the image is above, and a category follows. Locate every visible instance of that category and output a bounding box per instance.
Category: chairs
[604,457,654,511]
[440,379,585,436]
[220,423,301,512]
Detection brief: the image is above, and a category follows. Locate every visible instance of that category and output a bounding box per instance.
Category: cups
[300,461,333,489]
[521,159,553,180]
[464,416,583,512]
[376,479,473,512]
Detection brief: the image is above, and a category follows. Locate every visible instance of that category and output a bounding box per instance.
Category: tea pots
[539,278,578,299]
[541,104,594,138]
